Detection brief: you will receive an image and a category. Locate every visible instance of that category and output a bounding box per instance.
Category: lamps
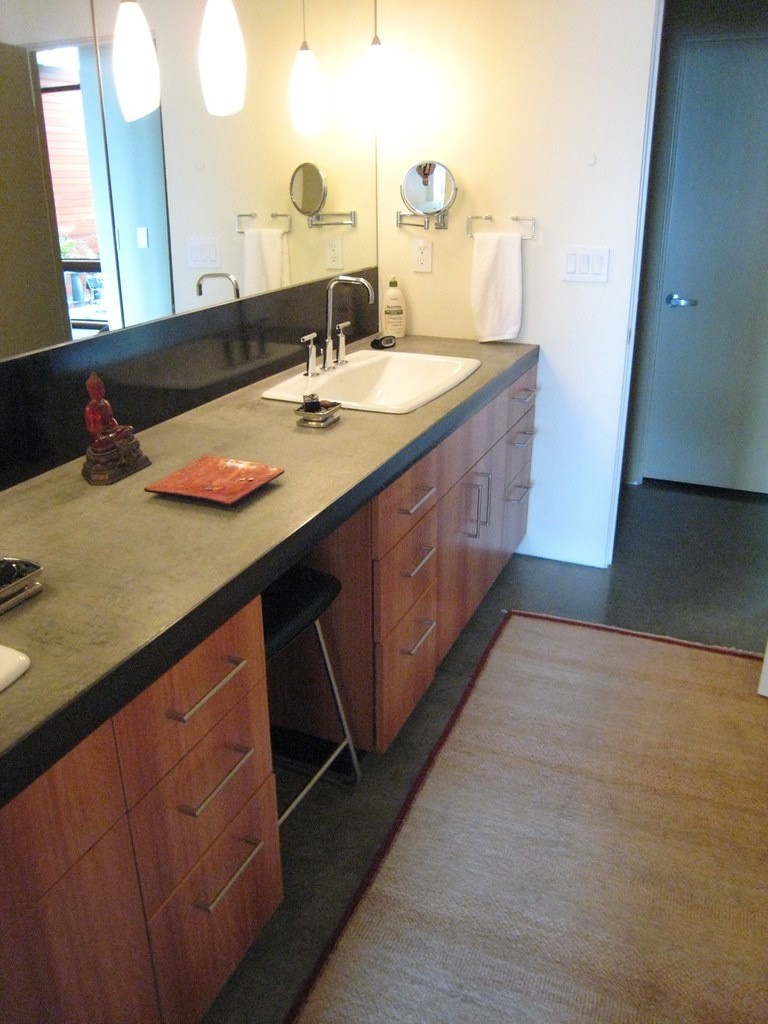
[1,0,381,368]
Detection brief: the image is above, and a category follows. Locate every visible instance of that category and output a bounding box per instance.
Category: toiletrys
[381,274,406,338]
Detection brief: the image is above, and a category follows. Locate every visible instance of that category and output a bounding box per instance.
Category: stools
[265,564,363,828]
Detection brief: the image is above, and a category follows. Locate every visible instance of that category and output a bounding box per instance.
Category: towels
[243,227,291,295]
[471,231,524,344]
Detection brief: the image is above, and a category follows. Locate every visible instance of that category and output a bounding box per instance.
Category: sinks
[260,347,482,415]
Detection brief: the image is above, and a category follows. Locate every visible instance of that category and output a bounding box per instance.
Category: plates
[145,456,285,505]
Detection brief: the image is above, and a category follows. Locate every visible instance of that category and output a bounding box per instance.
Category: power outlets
[412,240,433,272]
[324,240,342,269]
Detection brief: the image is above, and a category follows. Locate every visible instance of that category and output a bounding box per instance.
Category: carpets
[287,609,768,1023]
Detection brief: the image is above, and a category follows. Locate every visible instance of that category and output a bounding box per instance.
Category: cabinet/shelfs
[0,363,544,1023]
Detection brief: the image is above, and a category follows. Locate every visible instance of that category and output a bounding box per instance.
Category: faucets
[320,276,374,372]
[195,272,241,299]
